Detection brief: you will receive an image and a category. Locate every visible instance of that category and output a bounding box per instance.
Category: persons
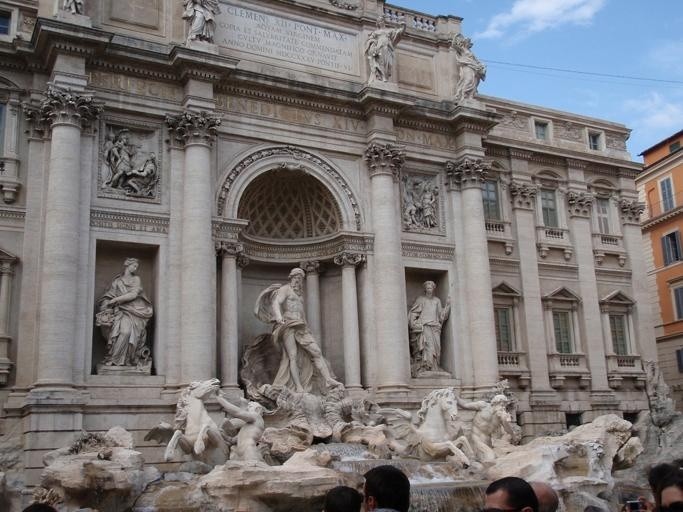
[363,464,410,512]
[321,485,361,512]
[405,180,439,229]
[483,476,559,512]
[585,458,683,512]
[452,32,486,102]
[96,258,155,367]
[408,280,453,370]
[271,266,343,394]
[103,133,158,195]
[216,389,265,462]
[182,0,221,43]
[456,394,515,461]
[365,16,407,83]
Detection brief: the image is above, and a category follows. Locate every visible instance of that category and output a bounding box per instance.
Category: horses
[164,377,230,467]
[409,388,476,467]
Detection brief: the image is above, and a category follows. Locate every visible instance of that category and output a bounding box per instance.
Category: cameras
[626,501,643,511]
